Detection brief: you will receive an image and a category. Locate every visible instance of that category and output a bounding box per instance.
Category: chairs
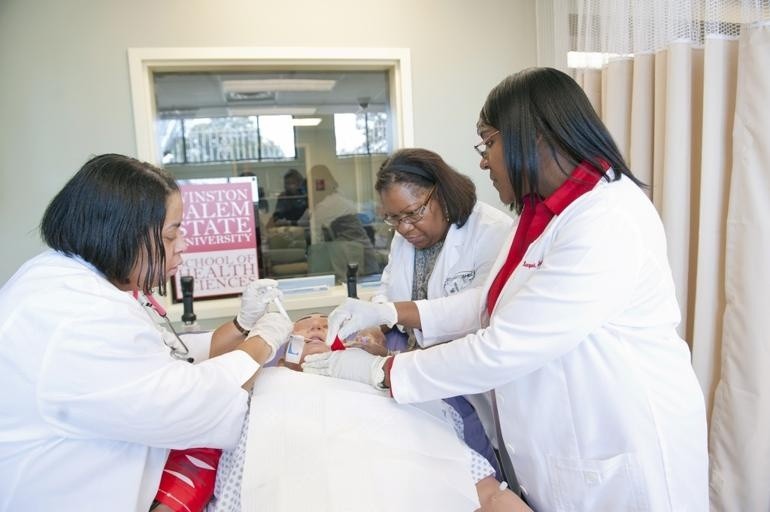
[308,240,363,286]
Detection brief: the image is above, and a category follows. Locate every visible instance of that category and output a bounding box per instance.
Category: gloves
[244,312,293,364]
[325,298,399,347]
[236,278,285,331]
[301,348,393,391]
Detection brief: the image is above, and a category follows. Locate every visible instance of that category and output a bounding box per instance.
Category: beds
[259,320,507,484]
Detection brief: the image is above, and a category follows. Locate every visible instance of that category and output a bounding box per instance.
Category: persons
[299,65,709,511]
[275,164,380,275]
[1,153,295,511]
[369,148,514,483]
[148,310,535,512]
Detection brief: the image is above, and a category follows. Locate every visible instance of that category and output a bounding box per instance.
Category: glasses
[474,130,501,157]
[384,184,436,227]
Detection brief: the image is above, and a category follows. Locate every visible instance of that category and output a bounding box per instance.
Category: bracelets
[232,315,251,336]
